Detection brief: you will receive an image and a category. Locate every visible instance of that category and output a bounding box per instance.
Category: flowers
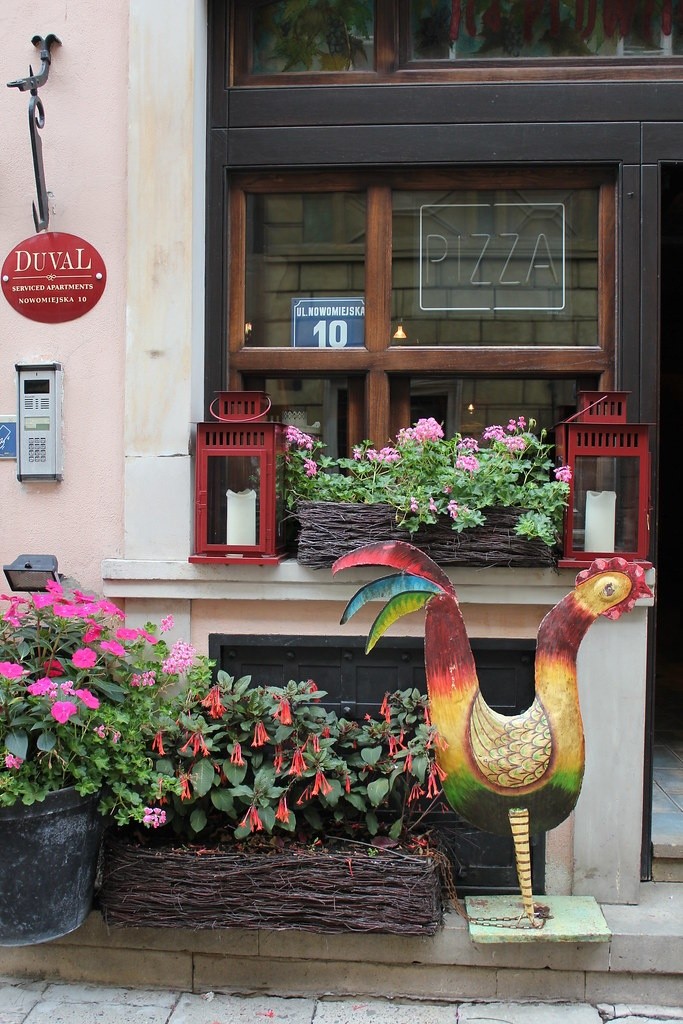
[118,671,448,848]
[281,417,573,549]
[0,580,201,828]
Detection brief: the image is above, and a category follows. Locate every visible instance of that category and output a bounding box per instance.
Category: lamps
[1,553,64,593]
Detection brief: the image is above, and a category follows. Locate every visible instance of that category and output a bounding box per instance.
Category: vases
[0,784,103,947]
